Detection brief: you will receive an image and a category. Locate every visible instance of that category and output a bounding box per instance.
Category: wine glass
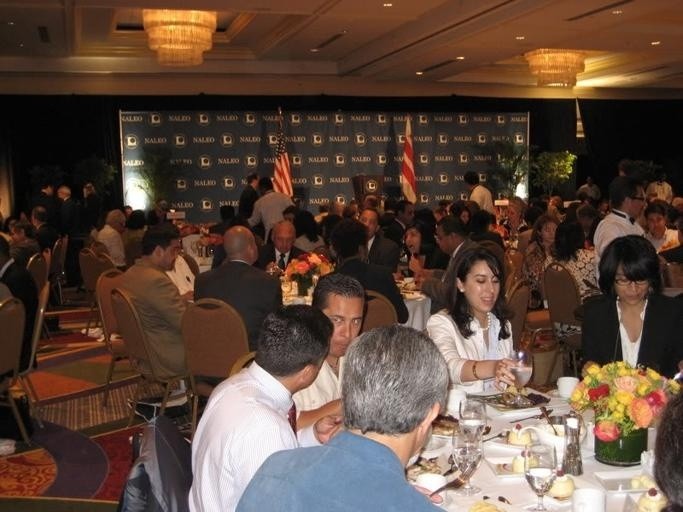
[452,425,481,495]
[460,399,487,450]
[525,443,558,511]
[280,277,292,303]
[507,351,533,407]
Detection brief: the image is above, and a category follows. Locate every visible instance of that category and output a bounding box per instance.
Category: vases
[593,407,648,465]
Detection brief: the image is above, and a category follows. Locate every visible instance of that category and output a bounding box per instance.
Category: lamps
[525,50,586,88]
[142,8,217,67]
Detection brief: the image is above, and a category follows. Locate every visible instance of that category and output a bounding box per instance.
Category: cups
[557,376,579,396]
[404,278,415,285]
[446,389,466,415]
[518,424,586,452]
[572,489,605,512]
[416,474,447,506]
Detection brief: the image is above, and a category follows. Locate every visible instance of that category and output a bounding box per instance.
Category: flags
[401,118,417,204]
[271,117,294,199]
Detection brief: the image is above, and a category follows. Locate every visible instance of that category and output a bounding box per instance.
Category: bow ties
[612,210,637,226]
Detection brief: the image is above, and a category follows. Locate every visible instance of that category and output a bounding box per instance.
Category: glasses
[613,276,650,287]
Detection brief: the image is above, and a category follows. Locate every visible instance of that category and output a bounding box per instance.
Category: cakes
[511,451,535,473]
[505,385,527,395]
[550,470,577,499]
[509,424,532,444]
[639,486,666,512]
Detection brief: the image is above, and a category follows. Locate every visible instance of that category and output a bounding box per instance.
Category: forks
[431,462,477,496]
[509,410,553,423]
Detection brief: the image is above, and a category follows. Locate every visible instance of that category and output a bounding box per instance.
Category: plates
[422,438,448,454]
[484,456,524,478]
[547,389,563,399]
[592,468,658,492]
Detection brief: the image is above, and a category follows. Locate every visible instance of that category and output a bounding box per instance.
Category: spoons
[482,430,510,442]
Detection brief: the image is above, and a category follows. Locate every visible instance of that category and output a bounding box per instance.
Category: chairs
[109,289,192,431]
[358,289,398,339]
[0,234,127,445]
[180,298,258,443]
[506,262,582,383]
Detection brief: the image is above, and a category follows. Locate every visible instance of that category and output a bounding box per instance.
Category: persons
[186,303,335,512]
[428,241,517,394]
[576,234,680,384]
[235,324,451,511]
[1,157,683,447]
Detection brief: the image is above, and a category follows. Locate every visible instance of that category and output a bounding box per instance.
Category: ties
[287,403,298,436]
[277,252,286,272]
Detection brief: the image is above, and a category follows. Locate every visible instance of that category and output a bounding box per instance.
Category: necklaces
[329,357,340,370]
[482,312,491,330]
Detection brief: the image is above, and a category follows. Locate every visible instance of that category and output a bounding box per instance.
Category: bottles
[562,417,583,476]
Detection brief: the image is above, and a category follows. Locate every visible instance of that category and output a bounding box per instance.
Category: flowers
[285,252,337,281]
[296,277,312,296]
[565,362,682,441]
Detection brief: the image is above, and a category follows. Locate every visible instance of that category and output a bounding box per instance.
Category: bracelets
[473,362,480,379]
